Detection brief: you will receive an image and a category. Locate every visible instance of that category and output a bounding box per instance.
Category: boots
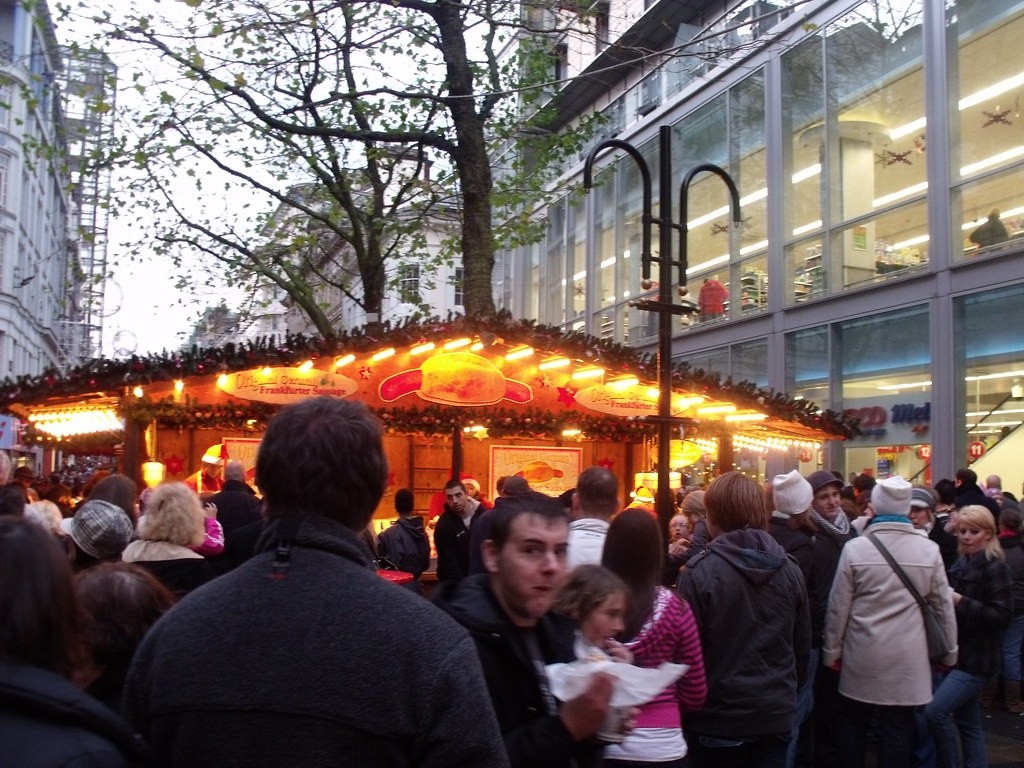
[981,673,1024,713]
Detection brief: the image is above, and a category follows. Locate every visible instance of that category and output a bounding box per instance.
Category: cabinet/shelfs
[600,252,822,341]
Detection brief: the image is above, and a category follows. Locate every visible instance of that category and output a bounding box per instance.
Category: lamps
[1012,378,1024,398]
[14,275,35,288]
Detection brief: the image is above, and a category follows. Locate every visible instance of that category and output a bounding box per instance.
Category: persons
[970,208,1009,253]
[0,395,1024,768]
[699,274,728,322]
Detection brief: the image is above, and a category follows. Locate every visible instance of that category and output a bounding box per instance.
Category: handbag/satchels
[924,604,953,665]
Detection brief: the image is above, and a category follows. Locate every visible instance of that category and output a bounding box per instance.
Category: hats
[808,470,843,494]
[911,487,936,508]
[629,485,655,504]
[934,479,956,503]
[59,499,133,559]
[502,477,529,496]
[682,491,706,518]
[202,444,229,466]
[14,467,35,480]
[458,471,480,492]
[559,488,578,508]
[772,470,814,516]
[36,475,47,483]
[871,475,912,517]
